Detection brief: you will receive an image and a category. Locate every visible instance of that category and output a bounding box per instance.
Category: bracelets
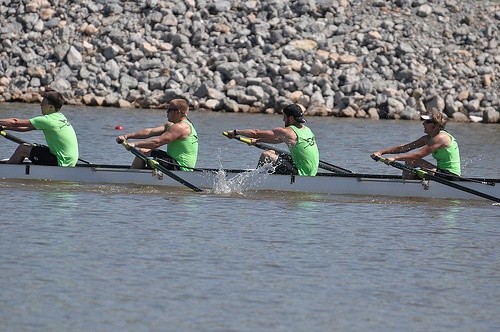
[234,129,238,135]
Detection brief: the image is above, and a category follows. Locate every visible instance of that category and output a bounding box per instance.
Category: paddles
[119,140,204,192]
[369,153,500,203]
[221,131,358,174]
[0,131,91,164]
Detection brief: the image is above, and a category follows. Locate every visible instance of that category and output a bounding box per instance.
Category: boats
[0,158,500,202]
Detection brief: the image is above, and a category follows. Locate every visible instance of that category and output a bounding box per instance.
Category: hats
[420,115,444,130]
[284,105,307,123]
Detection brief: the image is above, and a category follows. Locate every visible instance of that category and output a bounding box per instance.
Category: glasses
[166,106,178,114]
[425,119,435,125]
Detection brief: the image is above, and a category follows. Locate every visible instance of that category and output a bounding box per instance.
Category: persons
[116,99,198,171]
[372,109,461,178]
[0,92,79,167]
[228,104,319,176]
